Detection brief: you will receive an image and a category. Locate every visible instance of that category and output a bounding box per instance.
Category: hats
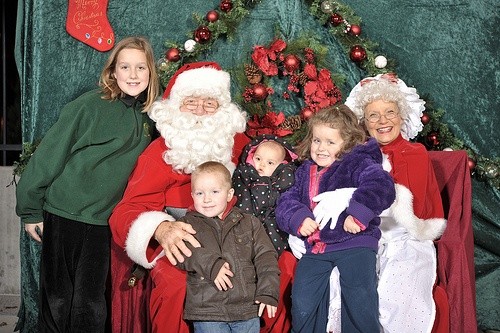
[159,62,231,102]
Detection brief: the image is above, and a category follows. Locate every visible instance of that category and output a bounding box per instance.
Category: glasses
[363,110,399,123]
[183,100,219,113]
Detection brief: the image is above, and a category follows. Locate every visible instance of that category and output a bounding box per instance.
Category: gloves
[288,234,306,260]
[310,190,349,232]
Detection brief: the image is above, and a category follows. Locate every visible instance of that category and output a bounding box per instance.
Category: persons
[166,161,280,333]
[232,138,297,264]
[107,61,252,332]
[273,103,396,333]
[14,36,167,333]
[345,70,447,333]
